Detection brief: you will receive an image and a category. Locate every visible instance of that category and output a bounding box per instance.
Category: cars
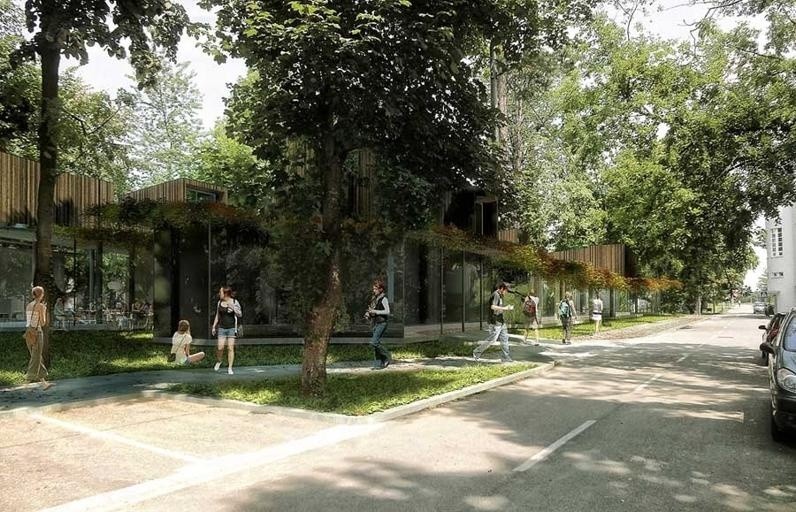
[752,301,796,445]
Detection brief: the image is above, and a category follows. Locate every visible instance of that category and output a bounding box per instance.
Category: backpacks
[523,299,536,317]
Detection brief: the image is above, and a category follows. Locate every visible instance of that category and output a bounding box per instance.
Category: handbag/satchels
[23,325,38,344]
[559,300,571,319]
[236,325,244,339]
[167,353,176,362]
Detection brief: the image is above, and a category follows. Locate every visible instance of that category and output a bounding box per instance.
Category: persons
[521,289,540,346]
[170,319,206,366]
[363,280,392,371]
[21,285,56,391]
[558,291,577,345]
[592,294,603,338]
[472,281,514,364]
[52,297,154,329]
[211,285,243,375]
